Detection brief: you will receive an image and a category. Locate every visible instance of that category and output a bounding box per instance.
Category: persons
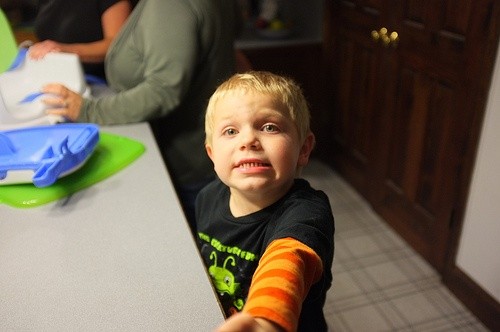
[42,0,226,211]
[19,0,130,86]
[192,70,335,332]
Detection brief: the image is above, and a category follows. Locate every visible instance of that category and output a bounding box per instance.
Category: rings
[65,101,69,108]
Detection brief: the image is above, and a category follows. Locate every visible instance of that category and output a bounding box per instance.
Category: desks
[0,122,227,332]
